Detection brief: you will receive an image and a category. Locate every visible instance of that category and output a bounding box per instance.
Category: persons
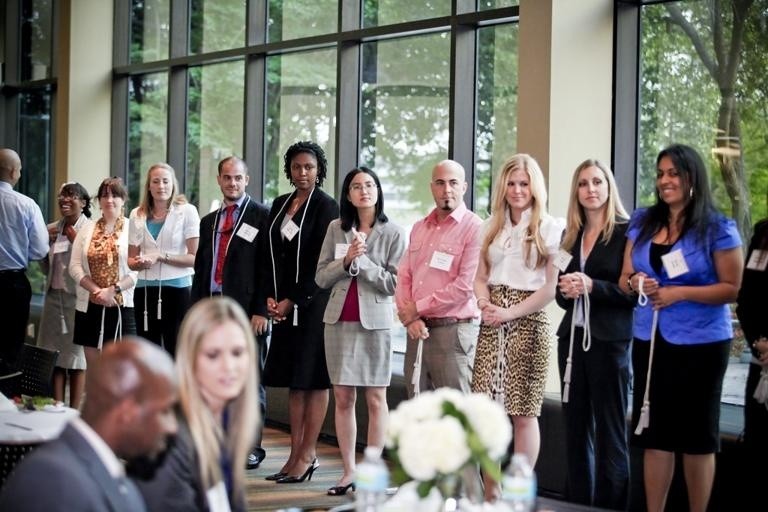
[393,161,486,402]
[620,146,746,512]
[558,160,631,508]
[128,163,200,352]
[2,331,182,512]
[37,182,96,410]
[474,153,562,479]
[314,165,403,499]
[129,297,261,512]
[68,177,139,370]
[0,148,50,402]
[733,218,768,510]
[191,155,273,469]
[266,142,339,483]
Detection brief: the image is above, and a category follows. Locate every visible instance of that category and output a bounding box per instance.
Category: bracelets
[165,253,169,264]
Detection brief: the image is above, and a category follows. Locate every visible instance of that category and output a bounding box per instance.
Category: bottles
[353,446,391,512]
[502,453,537,511]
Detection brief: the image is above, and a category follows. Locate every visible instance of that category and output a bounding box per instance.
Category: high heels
[328,482,356,495]
[276,457,320,483]
[265,471,288,481]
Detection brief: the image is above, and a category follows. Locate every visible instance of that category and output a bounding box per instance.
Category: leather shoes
[245,452,260,470]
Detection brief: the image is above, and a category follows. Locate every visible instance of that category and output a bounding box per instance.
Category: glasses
[56,193,81,201]
[349,182,377,190]
